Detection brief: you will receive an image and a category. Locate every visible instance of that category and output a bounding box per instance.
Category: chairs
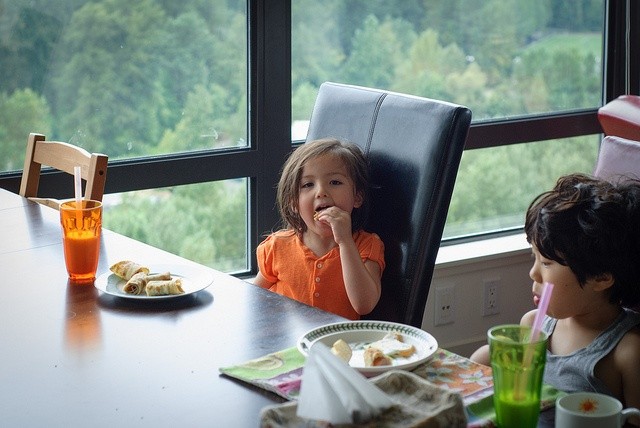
[233,79,473,326]
[591,136,640,191]
[593,91,640,191]
[20,132,109,223]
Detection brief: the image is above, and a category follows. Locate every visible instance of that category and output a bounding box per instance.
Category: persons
[470,173,640,426]
[252,137,386,322]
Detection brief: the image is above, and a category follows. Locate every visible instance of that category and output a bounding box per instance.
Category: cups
[487,324,548,428]
[555,392,640,428]
[60,200,103,280]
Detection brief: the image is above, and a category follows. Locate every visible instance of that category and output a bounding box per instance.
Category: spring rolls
[363,347,391,366]
[122,271,148,296]
[145,277,186,296]
[108,259,149,281]
[145,271,171,283]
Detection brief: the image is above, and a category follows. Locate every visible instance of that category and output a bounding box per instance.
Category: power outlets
[433,283,458,325]
[478,275,502,318]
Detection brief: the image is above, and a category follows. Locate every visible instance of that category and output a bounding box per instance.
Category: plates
[297,320,438,378]
[94,264,214,300]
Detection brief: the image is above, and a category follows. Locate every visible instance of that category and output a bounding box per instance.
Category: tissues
[257,339,471,428]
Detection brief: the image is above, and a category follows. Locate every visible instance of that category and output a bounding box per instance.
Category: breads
[367,330,415,362]
